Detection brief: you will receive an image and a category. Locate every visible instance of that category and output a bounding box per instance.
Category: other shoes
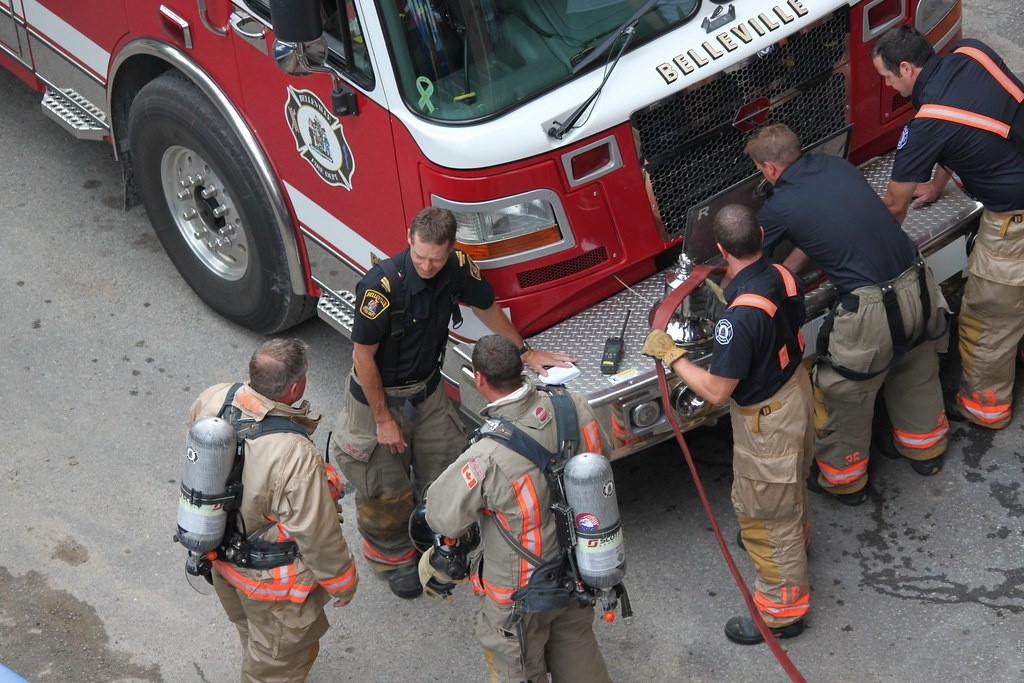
[875,426,943,476]
[945,396,967,421]
[385,563,423,599]
[805,469,868,505]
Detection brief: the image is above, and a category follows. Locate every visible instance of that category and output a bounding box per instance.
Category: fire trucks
[0,0,983,471]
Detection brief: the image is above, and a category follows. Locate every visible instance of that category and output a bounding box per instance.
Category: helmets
[408,499,480,555]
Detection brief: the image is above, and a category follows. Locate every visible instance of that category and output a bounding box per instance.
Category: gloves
[642,329,689,370]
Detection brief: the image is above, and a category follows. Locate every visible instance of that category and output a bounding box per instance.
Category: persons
[186,339,359,683]
[641,202,817,645]
[330,207,579,599]
[425,335,610,683]
[721,125,953,506]
[872,22,1023,430]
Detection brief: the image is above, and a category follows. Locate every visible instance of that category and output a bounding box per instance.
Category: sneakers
[724,612,806,645]
[737,528,812,554]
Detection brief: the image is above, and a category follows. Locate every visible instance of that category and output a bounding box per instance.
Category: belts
[350,363,442,408]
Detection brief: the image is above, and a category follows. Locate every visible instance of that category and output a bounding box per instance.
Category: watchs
[520,339,531,354]
